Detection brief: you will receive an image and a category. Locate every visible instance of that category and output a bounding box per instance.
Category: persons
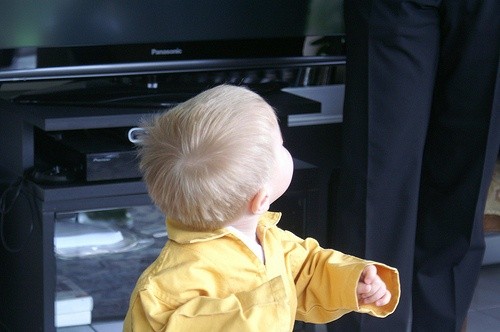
[333,0,500,332]
[122,84,401,332]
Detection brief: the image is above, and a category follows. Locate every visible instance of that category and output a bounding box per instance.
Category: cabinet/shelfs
[4,32,364,332]
